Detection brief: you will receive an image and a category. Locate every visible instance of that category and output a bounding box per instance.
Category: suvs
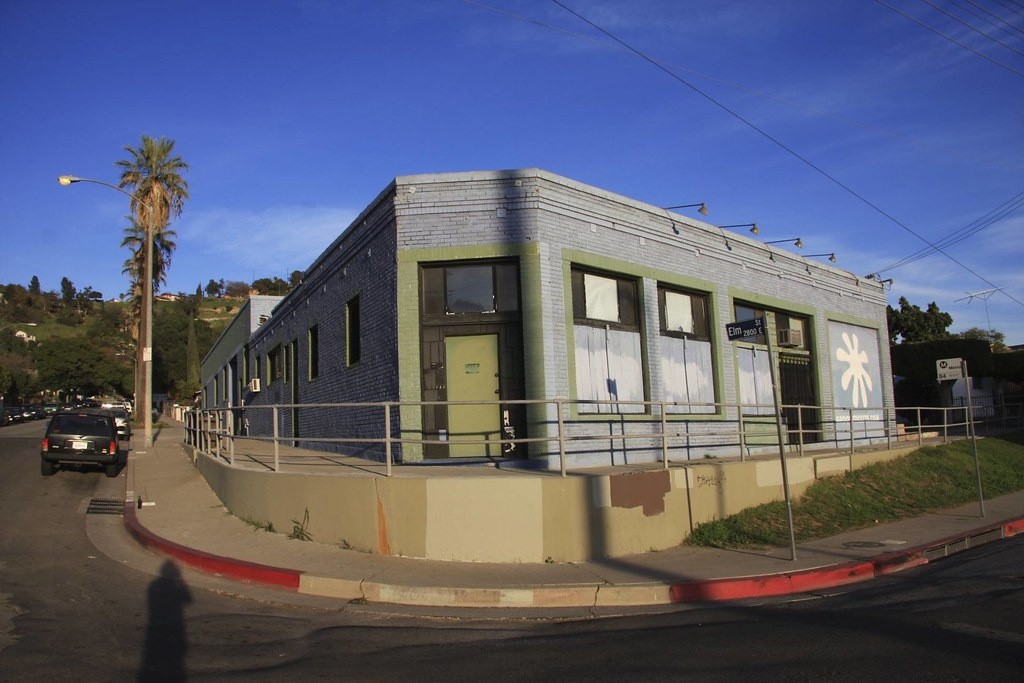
[40,410,120,476]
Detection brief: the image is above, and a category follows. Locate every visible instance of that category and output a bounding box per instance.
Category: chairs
[95,421,106,435]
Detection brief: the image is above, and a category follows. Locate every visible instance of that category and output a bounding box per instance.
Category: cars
[0,399,133,426]
[100,408,131,441]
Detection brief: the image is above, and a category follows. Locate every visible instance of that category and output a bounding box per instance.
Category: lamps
[718,223,759,235]
[802,253,835,263]
[764,237,802,247]
[662,202,708,215]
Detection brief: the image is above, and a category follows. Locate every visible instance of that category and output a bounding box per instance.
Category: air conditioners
[249,378,261,393]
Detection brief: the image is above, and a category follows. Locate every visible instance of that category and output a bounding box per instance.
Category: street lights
[115,353,136,417]
[57,174,153,447]
